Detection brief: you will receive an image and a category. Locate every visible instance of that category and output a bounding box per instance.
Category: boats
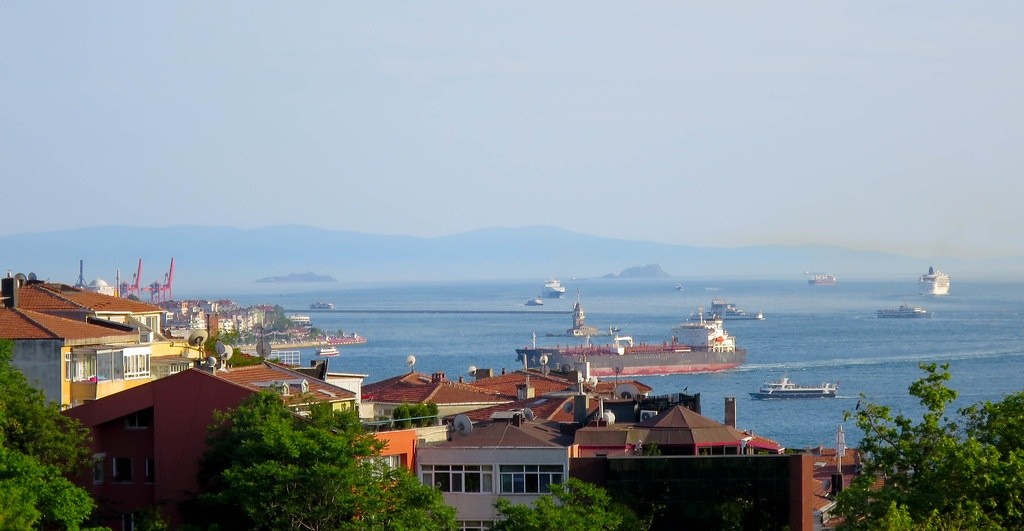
[917,267,951,295]
[290,313,311,324]
[542,278,566,298]
[524,296,543,306]
[808,272,837,286]
[515,305,748,379]
[309,299,334,309]
[314,345,340,356]
[675,284,684,292]
[876,306,934,318]
[748,377,839,401]
[688,298,765,320]
[566,289,612,338]
